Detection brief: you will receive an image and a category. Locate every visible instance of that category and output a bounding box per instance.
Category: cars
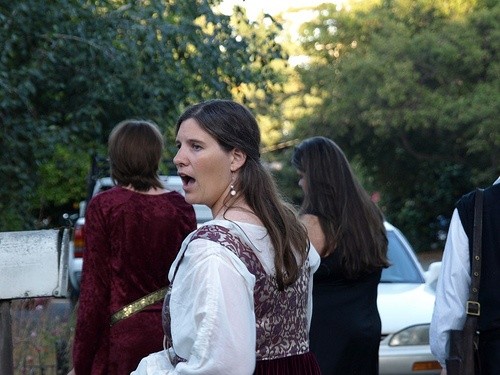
[64,175,213,306]
[373,223,448,375]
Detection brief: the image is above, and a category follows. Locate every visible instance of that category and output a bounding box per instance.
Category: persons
[291,136,392,375]
[65,120,197,375]
[128,100,321,375]
[429,175,500,375]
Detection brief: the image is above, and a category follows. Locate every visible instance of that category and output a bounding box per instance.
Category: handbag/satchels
[446,329,481,375]
